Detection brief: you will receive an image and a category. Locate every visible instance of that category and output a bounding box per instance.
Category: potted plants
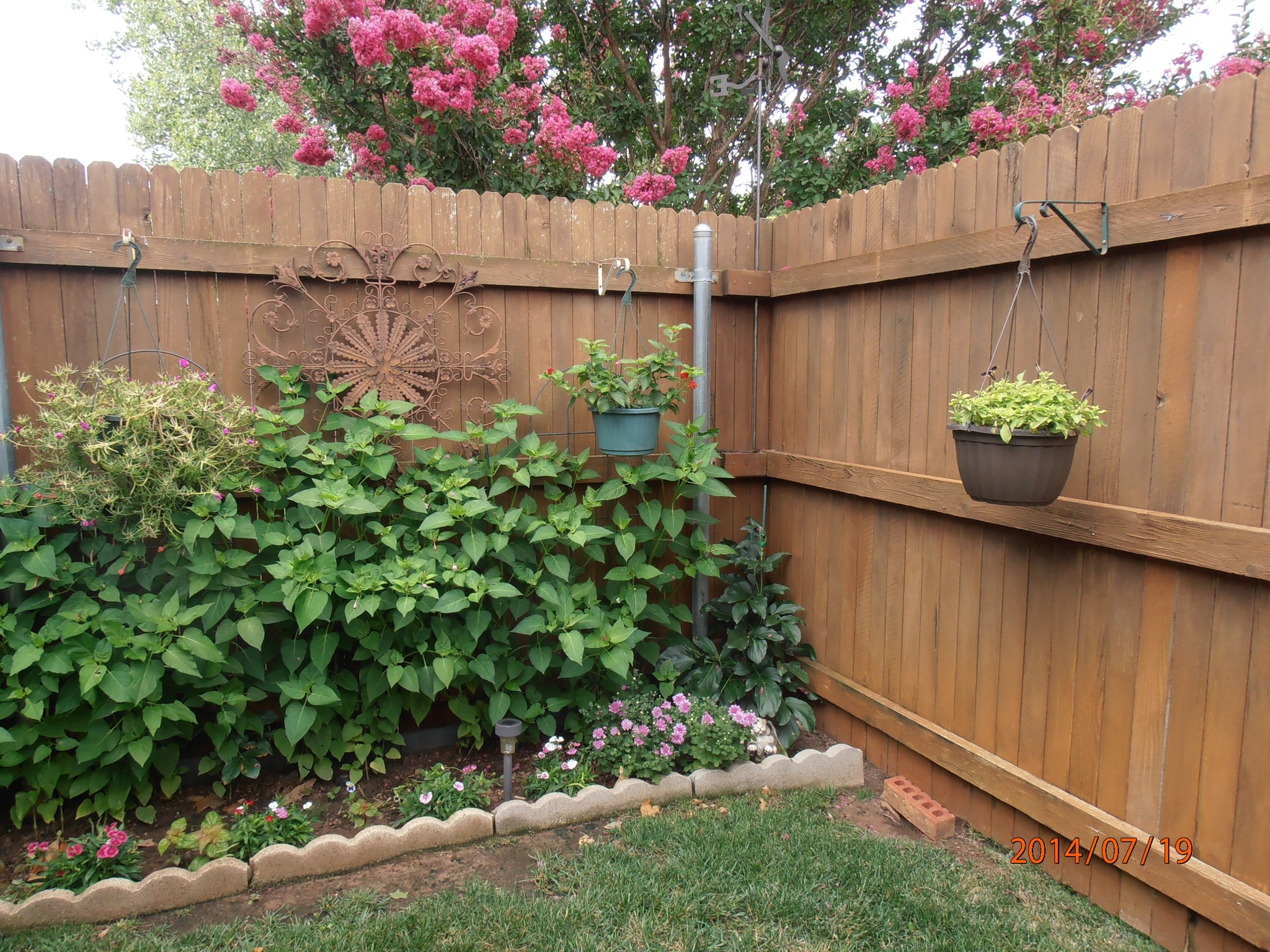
[949,373,1109,507]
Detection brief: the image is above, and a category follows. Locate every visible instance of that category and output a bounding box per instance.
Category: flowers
[540,319,702,408]
[4,357,265,526]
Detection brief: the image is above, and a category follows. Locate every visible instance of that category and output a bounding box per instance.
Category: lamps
[495,717,522,755]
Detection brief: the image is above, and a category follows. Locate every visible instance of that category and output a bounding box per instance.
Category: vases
[96,412,197,471]
[592,402,664,455]
